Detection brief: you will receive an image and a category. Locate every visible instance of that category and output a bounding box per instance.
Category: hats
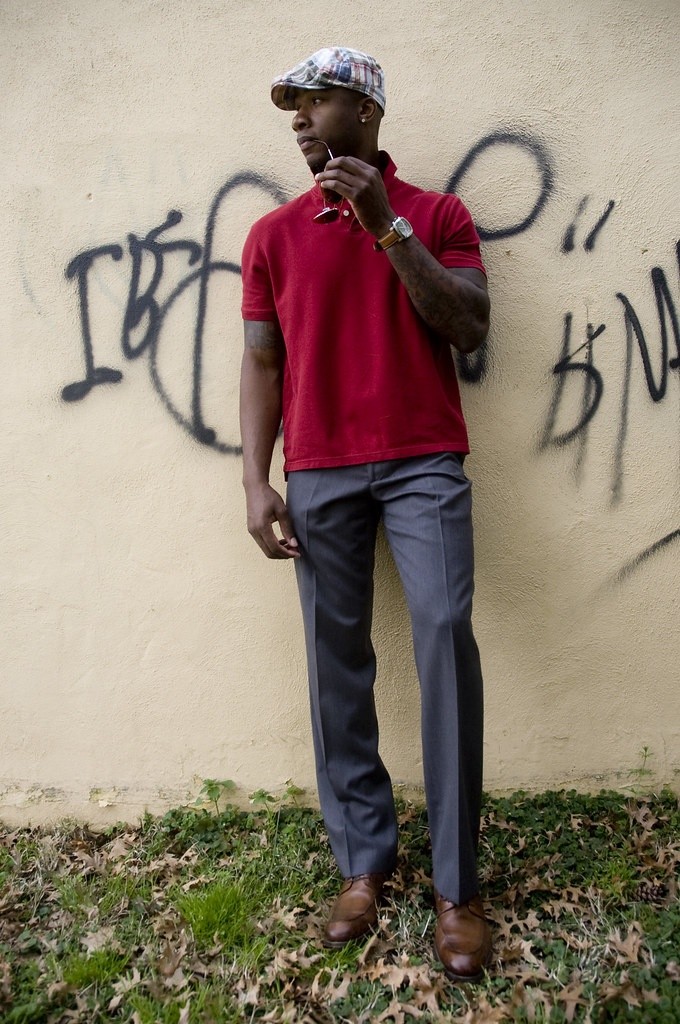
[270,47,386,116]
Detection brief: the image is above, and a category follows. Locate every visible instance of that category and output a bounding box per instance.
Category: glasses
[311,139,343,223]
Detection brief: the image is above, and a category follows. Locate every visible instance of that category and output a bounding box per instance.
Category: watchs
[373,216,413,253]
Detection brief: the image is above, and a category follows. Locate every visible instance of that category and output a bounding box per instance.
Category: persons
[240,48,491,982]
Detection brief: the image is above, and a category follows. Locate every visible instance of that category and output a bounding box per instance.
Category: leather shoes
[324,873,381,947]
[433,889,491,983]
